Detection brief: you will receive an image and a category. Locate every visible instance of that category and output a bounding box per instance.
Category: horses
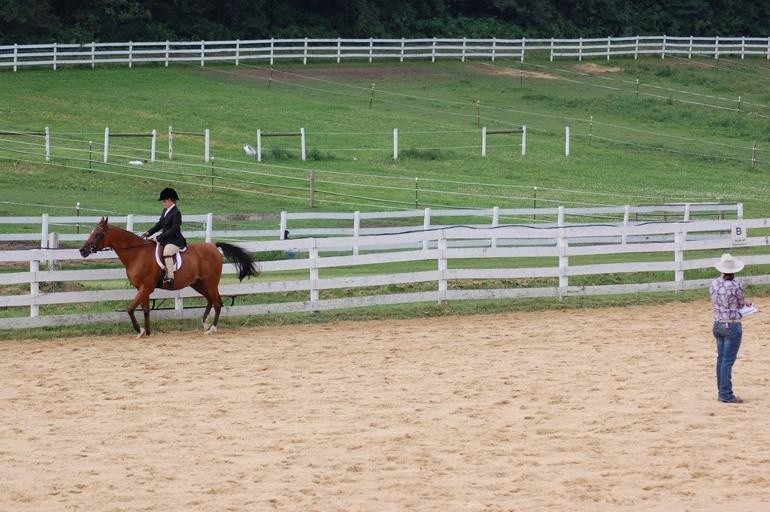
[78,216,259,339]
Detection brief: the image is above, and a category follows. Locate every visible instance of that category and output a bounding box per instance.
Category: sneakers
[164,278,173,288]
[719,396,743,402]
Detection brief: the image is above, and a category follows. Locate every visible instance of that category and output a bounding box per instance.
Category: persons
[708,254,753,404]
[143,188,186,289]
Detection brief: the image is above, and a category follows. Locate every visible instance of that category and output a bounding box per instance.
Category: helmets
[158,187,179,200]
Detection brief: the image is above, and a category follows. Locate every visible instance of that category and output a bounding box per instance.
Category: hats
[713,253,744,273]
